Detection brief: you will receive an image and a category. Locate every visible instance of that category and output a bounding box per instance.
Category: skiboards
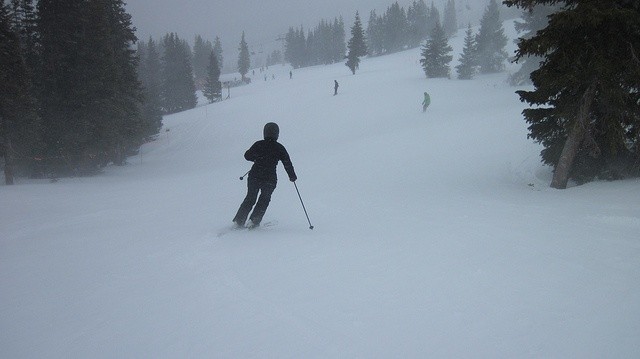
[214,219,279,238]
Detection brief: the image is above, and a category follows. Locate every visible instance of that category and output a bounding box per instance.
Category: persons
[232,123,315,230]
[333,79,339,95]
[421,92,431,112]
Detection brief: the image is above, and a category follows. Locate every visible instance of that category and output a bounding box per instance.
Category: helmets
[264,122,279,141]
